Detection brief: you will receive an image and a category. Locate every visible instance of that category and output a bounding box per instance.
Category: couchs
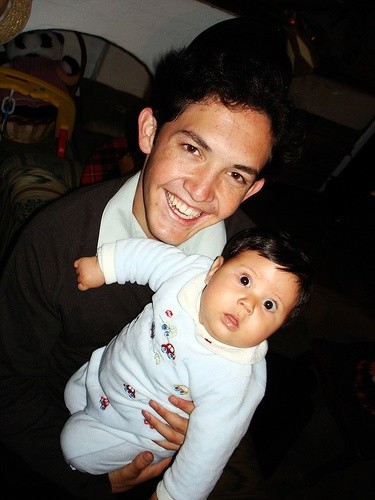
[0,137,150,265]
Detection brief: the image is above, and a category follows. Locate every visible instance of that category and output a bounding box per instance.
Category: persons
[55,220,320,500]
[0,39,337,500]
